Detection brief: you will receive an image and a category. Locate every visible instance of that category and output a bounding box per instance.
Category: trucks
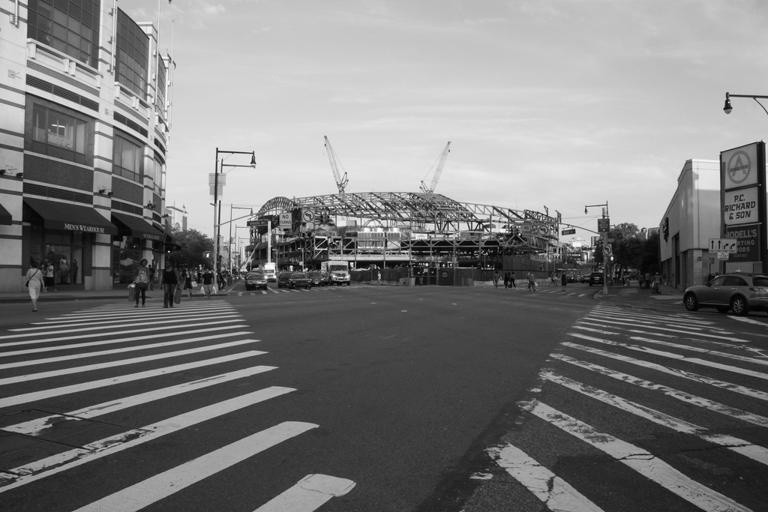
[321,260,351,287]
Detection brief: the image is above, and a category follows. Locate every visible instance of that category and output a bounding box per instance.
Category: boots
[134,304,145,308]
[160,306,176,308]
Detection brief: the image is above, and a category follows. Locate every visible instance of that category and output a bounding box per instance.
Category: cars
[244,262,327,291]
[682,272,768,317]
[582,271,604,287]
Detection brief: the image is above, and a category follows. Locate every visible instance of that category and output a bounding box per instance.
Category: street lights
[212,147,256,295]
[584,200,612,296]
[723,91,768,115]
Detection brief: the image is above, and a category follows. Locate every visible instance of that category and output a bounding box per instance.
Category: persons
[42,256,80,293]
[25,261,45,311]
[133,258,148,308]
[181,267,232,297]
[160,259,181,308]
[492,270,536,294]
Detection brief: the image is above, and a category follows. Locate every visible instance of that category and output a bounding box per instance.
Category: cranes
[419,140,452,194]
[323,134,349,194]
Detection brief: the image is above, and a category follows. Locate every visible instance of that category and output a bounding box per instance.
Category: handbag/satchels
[200,286,205,294]
[183,283,187,290]
[191,281,197,288]
[210,286,216,295]
[25,280,29,287]
[174,288,182,304]
